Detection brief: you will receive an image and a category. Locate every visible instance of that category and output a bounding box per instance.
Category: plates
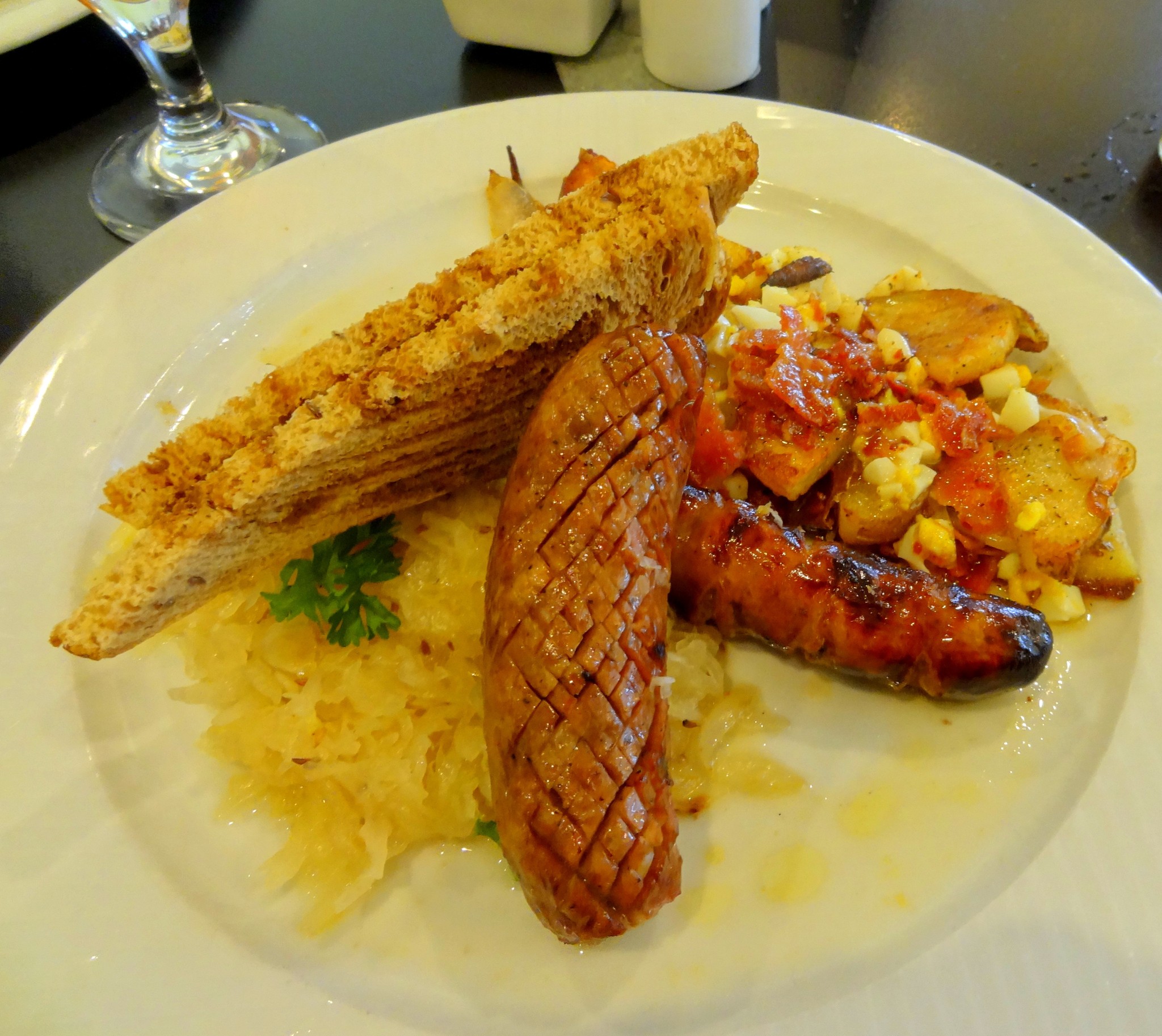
[0,91,1160,1036]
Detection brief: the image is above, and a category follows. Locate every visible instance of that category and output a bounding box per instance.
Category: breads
[46,123,763,666]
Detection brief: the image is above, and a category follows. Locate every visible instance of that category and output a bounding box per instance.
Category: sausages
[481,322,1058,948]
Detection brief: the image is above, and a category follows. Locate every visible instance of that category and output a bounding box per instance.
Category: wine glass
[78,0,328,245]
